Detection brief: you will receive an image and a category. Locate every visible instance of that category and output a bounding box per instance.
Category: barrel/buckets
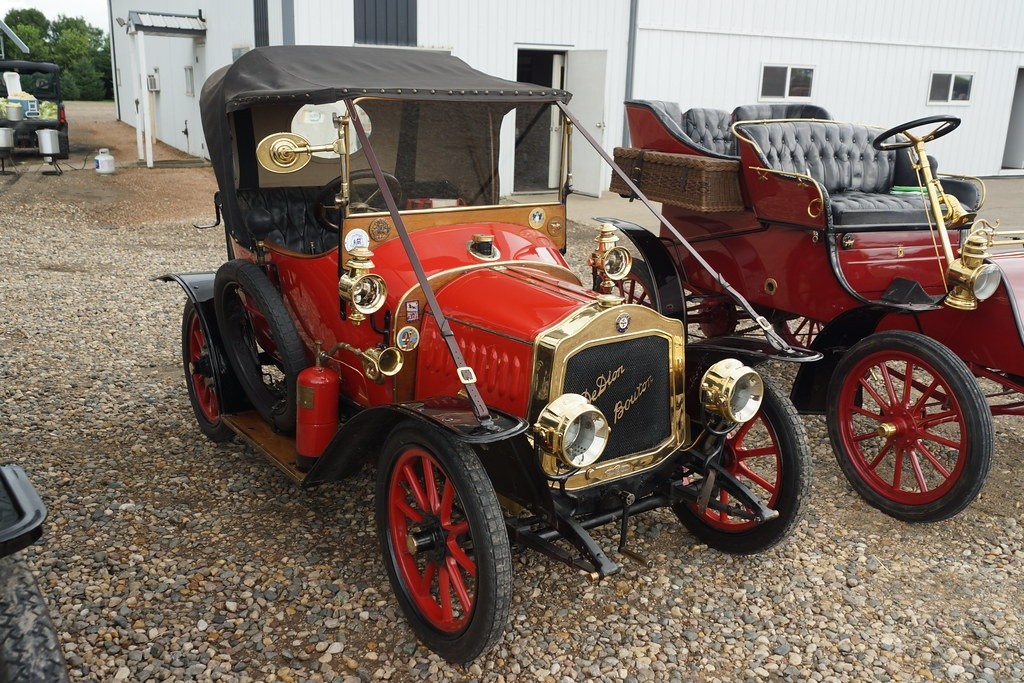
[37,129,60,154]
[6,106,22,121]
[0,128,14,147]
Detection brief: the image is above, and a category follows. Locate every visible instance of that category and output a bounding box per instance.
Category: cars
[150,44,813,665]
[610,98,1024,524]
[0,61,72,160]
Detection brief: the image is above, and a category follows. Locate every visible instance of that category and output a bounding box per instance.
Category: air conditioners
[147,74,160,91]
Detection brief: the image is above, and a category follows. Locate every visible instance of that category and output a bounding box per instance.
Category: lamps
[116,17,126,26]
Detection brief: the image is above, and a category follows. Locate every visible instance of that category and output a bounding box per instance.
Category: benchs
[731,119,986,232]
[677,105,833,159]
[242,181,454,255]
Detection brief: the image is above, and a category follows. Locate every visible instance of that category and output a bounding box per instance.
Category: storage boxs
[3,71,40,118]
